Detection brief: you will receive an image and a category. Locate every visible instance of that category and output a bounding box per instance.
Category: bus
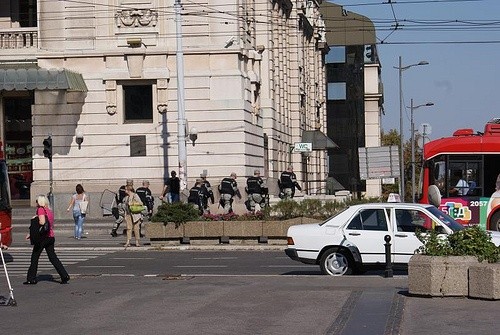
[0,137,15,251]
[402,132,500,231]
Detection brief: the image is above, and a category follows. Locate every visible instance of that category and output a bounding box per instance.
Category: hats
[195,178,205,183]
[171,171,176,175]
[231,172,236,179]
[143,180,150,185]
[254,170,260,173]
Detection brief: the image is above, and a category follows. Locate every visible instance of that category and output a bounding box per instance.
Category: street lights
[394,53,435,211]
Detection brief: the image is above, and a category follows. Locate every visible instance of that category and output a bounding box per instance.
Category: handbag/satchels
[79,194,89,214]
[129,192,146,214]
[36,206,50,235]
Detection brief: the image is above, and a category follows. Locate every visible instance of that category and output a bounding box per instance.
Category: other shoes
[136,243,142,246]
[23,280,37,284]
[73,235,78,238]
[60,275,70,284]
[78,238,81,239]
[111,229,117,237]
[122,242,131,246]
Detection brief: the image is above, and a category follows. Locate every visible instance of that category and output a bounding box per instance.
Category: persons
[401,212,431,232]
[245,169,269,214]
[65,184,89,240]
[277,165,302,202]
[188,178,208,218]
[199,174,215,215]
[448,173,470,196]
[121,185,143,248]
[159,170,180,204]
[22,194,71,286]
[108,179,137,237]
[136,180,154,222]
[218,172,242,215]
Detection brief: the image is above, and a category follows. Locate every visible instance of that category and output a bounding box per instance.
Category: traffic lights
[40,132,55,218]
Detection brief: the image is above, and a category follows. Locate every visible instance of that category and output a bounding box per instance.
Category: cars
[283,192,500,277]
